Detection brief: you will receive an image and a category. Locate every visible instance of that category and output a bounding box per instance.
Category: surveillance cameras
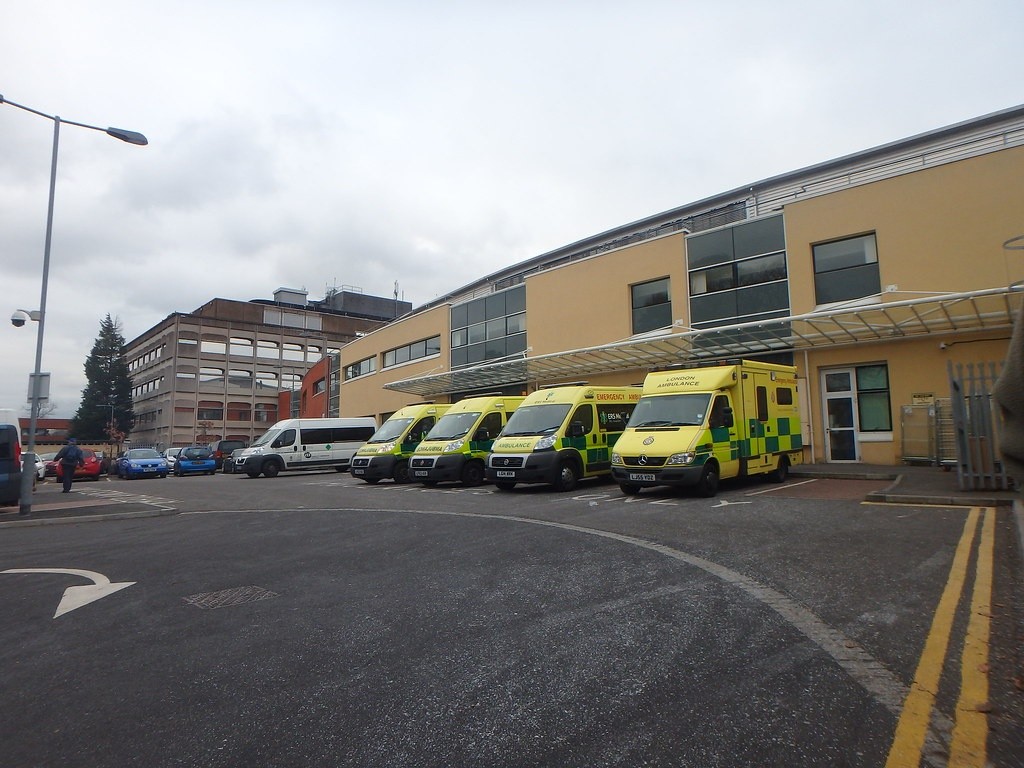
[940,342,947,350]
[11,312,26,327]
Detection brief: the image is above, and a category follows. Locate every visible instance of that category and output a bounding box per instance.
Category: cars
[115,448,170,480]
[223,449,248,472]
[162,448,183,473]
[56,448,101,482]
[173,445,217,477]
[95,450,111,475]
[41,452,59,478]
[22,449,46,481]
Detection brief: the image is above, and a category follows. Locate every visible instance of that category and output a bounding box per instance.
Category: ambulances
[408,395,526,486]
[609,359,805,497]
[485,386,643,492]
[349,403,455,484]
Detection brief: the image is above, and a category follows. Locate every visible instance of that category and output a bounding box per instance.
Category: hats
[68,438,77,444]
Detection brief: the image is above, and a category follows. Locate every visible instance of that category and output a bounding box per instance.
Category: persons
[54,438,84,492]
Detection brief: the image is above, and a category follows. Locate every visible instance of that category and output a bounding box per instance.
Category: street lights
[0,94,150,515]
[95,403,114,474]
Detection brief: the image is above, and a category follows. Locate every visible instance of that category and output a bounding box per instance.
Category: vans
[235,417,379,479]
[0,409,22,509]
[208,439,247,468]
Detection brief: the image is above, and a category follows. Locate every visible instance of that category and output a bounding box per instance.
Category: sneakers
[62,489,71,493]
